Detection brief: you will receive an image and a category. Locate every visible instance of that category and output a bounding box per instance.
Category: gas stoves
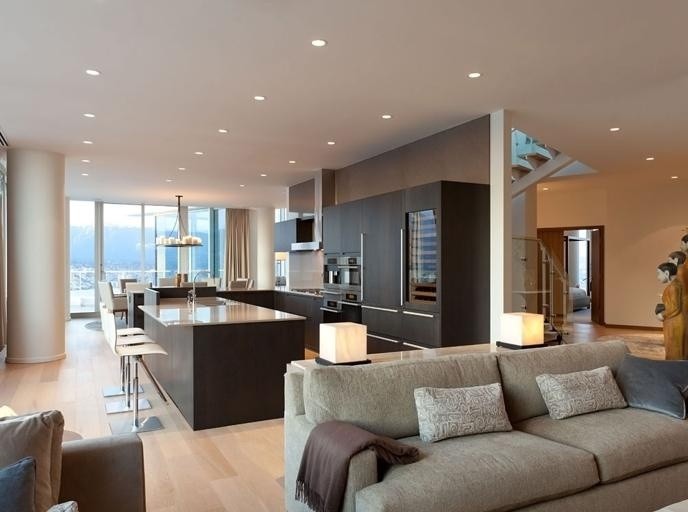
[292,288,323,294]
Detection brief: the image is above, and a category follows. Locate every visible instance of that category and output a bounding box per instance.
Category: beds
[563,236,590,310]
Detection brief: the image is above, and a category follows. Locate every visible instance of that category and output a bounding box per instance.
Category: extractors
[289,168,334,252]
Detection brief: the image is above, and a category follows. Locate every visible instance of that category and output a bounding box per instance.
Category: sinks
[191,298,227,307]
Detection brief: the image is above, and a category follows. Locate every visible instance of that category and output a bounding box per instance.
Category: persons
[667,250,688,360]
[656,263,686,360]
[679,234,688,297]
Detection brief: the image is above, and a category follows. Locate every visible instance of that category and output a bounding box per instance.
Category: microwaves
[323,265,360,290]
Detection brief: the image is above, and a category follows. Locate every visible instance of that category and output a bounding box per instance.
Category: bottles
[175,273,187,287]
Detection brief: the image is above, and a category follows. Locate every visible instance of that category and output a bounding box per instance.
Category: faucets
[191,270,210,304]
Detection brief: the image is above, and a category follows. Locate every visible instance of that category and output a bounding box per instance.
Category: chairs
[102,303,144,396]
[96,275,257,323]
[107,313,170,438]
[105,313,155,416]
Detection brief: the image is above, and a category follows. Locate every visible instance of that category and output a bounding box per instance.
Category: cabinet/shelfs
[274,290,323,354]
[217,292,273,309]
[320,198,362,259]
[126,292,145,351]
[274,276,285,292]
[361,179,490,354]
[272,218,311,254]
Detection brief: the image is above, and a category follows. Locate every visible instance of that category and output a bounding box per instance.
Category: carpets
[594,334,668,360]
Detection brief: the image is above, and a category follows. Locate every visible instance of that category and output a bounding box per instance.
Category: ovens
[317,291,361,324]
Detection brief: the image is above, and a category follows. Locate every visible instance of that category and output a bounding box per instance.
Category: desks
[282,342,506,370]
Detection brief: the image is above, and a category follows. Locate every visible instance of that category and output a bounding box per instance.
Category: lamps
[274,250,289,261]
[495,312,547,353]
[315,318,373,368]
[153,194,204,248]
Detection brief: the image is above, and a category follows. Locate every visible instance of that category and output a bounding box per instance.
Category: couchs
[270,340,687,512]
[42,426,155,512]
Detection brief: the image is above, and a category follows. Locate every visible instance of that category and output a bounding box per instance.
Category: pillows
[47,500,79,512]
[413,383,513,442]
[535,366,626,422]
[617,353,687,420]
[0,454,44,511]
[0,412,67,512]
